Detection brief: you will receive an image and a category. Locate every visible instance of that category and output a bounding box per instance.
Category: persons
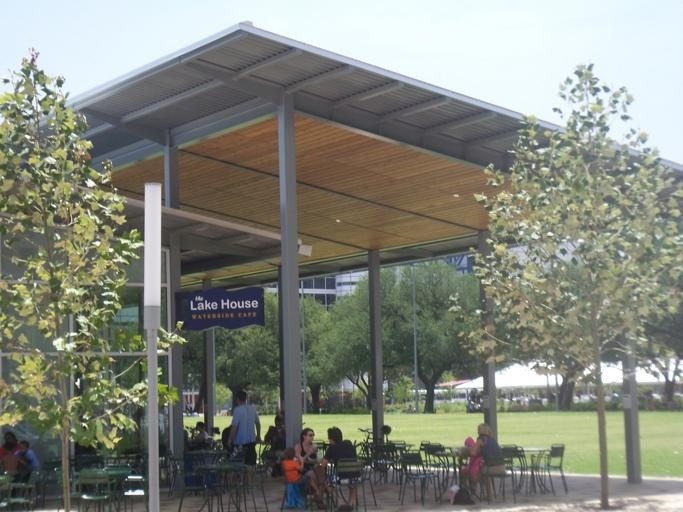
[467,389,620,413]
[195,391,361,512]
[468,423,504,503]
[0,431,41,497]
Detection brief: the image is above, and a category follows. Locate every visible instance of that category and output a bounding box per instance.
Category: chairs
[0,425,571,511]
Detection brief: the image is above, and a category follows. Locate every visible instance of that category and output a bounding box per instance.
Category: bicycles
[185,426,224,462]
[355,426,403,471]
